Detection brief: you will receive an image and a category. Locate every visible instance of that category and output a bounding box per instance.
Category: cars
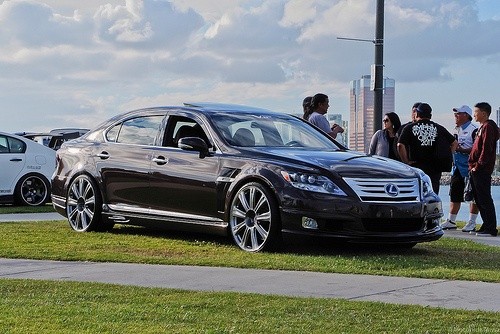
[12,126,91,152]
[49,103,445,254]
[0,131,57,207]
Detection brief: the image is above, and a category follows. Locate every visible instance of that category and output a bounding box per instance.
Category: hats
[414,103,432,113]
[453,105,473,119]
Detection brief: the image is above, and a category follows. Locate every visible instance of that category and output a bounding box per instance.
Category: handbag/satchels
[463,172,475,204]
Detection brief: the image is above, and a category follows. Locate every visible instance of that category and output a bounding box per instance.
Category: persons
[302,93,341,139]
[368,102,457,195]
[440,105,479,232]
[468,102,500,236]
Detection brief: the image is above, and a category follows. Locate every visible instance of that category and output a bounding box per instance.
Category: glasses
[454,112,459,115]
[382,118,389,123]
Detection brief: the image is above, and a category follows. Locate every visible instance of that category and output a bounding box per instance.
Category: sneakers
[439,219,458,230]
[462,223,477,232]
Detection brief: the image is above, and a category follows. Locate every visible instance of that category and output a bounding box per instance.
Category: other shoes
[470,230,498,237]
